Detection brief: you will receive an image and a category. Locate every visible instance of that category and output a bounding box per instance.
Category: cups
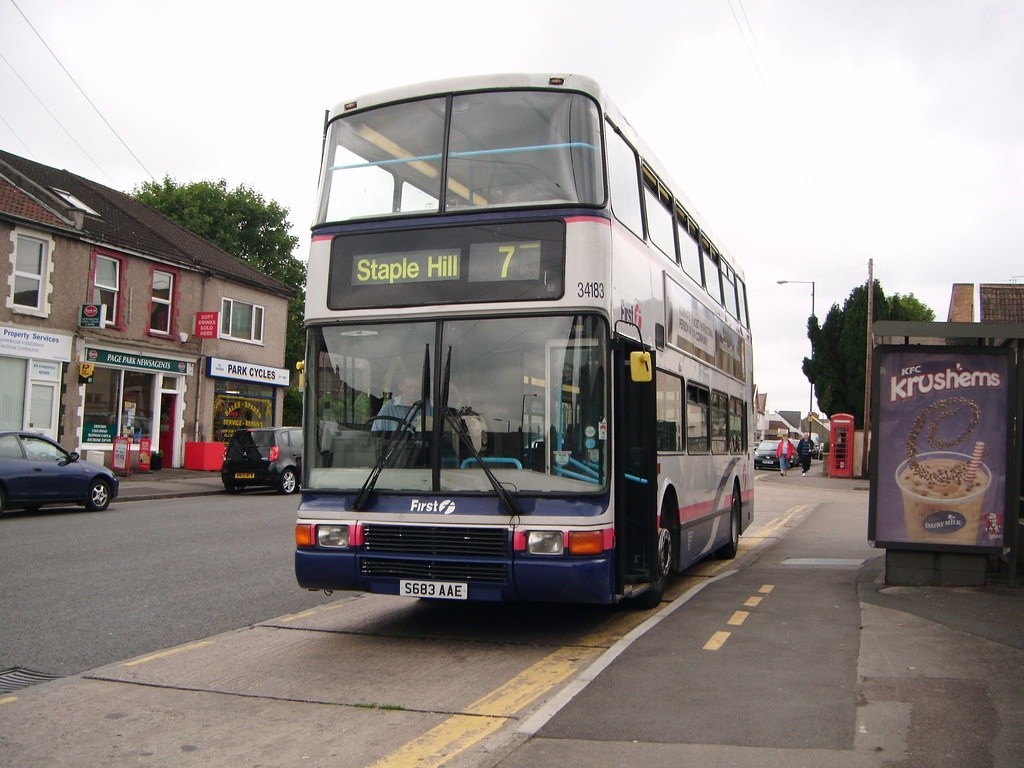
[894,450,993,547]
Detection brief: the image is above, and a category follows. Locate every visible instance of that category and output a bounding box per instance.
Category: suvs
[220,426,301,494]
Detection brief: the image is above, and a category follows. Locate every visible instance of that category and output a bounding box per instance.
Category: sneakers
[780,469,786,477]
[801,473,806,477]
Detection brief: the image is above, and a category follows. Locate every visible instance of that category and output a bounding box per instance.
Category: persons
[776,434,793,475]
[796,431,814,477]
[371,372,434,448]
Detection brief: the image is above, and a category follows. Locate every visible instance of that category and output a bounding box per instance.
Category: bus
[288,71,759,621]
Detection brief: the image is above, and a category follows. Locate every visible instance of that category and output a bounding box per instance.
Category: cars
[787,438,824,460]
[752,439,796,471]
[0,431,120,518]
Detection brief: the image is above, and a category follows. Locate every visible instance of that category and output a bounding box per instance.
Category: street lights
[776,280,815,441]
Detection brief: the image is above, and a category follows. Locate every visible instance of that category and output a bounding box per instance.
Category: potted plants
[151,450,164,470]
